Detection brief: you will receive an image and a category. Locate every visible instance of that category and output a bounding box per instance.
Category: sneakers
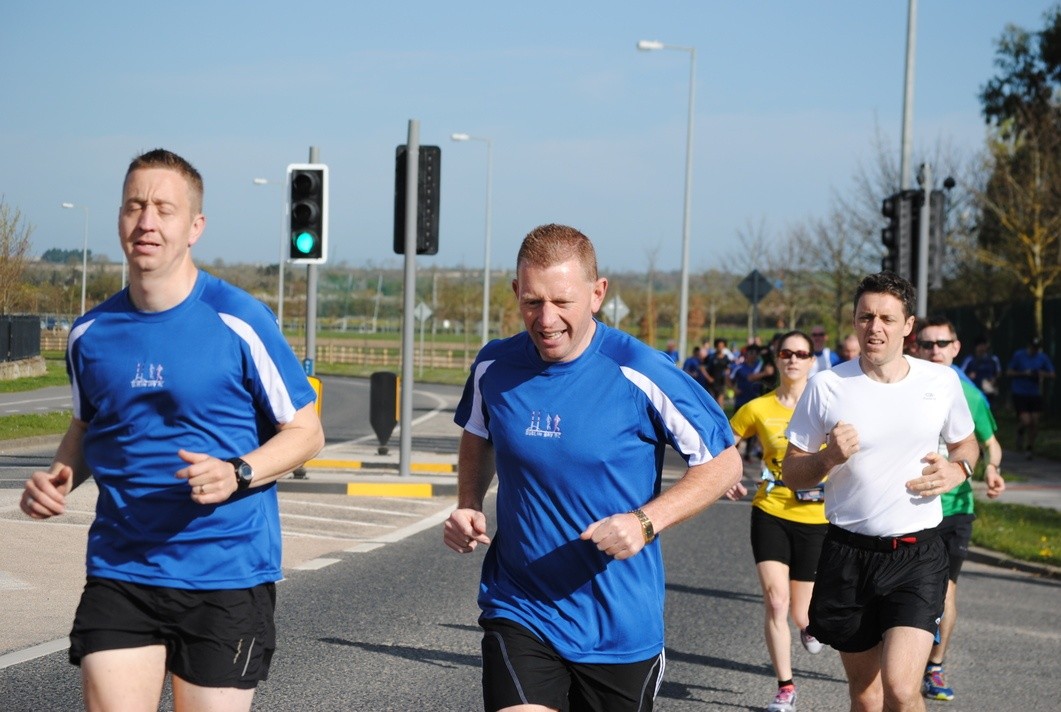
[768,684,798,712]
[920,668,955,703]
[800,629,826,655]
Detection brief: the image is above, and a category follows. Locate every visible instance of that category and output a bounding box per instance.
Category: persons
[659,332,1057,481]
[441,224,746,709]
[899,322,1006,704]
[717,330,835,712]
[18,149,326,712]
[777,272,981,711]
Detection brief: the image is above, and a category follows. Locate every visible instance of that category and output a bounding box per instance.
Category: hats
[811,332,826,337]
[1028,337,1043,351]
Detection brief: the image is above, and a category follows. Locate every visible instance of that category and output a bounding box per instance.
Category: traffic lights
[288,165,326,262]
[880,196,900,274]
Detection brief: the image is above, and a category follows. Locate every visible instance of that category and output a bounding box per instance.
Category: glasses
[915,339,956,350]
[776,349,813,361]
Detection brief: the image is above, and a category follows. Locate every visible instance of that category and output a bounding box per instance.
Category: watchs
[224,456,254,493]
[627,508,655,543]
